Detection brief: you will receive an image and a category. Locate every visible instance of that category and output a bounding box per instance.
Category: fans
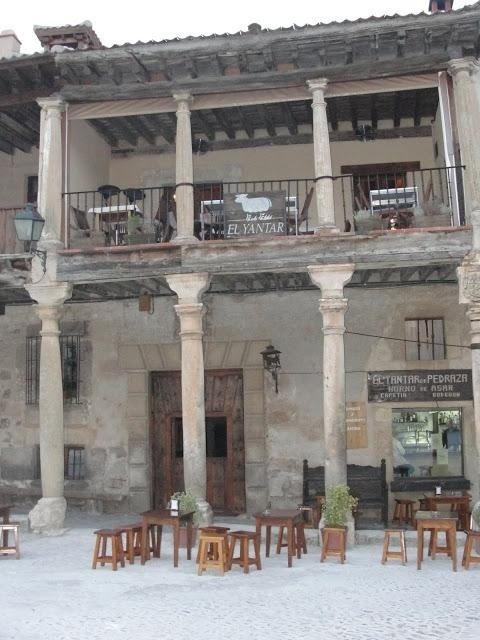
[192,138,208,155]
[356,122,376,143]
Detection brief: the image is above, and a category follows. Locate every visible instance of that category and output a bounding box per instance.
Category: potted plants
[319,485,357,553]
[167,491,201,548]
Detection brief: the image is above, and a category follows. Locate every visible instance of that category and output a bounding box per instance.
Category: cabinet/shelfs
[393,420,433,455]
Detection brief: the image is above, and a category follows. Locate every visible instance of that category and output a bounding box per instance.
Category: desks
[414,510,458,573]
[253,509,302,568]
[138,508,195,568]
[0,503,13,554]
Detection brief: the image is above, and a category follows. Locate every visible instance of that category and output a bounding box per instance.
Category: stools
[199,526,230,576]
[382,526,408,564]
[277,520,308,553]
[228,531,262,573]
[394,467,409,477]
[0,521,21,559]
[321,526,348,564]
[462,529,480,568]
[418,464,434,477]
[94,521,156,570]
[296,487,478,530]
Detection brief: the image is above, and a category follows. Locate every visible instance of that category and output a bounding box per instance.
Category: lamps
[258,343,282,393]
[12,202,46,270]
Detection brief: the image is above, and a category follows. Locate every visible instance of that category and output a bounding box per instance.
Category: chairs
[70,202,165,241]
[165,187,314,241]
[352,179,434,229]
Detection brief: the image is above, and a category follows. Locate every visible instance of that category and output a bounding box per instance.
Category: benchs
[303,459,388,527]
